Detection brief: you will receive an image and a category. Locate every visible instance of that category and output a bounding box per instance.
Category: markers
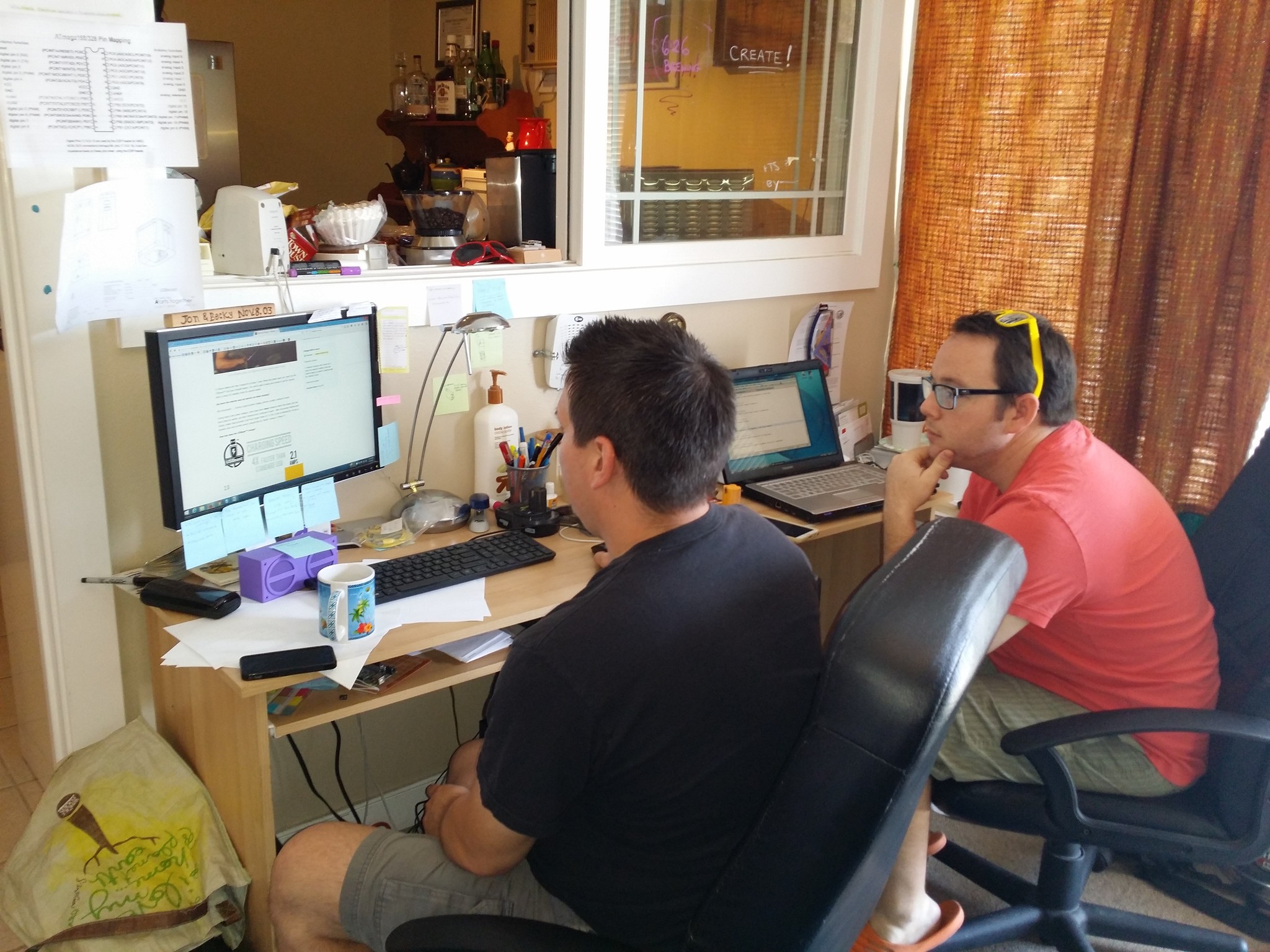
[289,266,361,277]
[81,578,163,586]
[499,441,514,467]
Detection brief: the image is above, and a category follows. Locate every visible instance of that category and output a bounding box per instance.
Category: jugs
[516,118,552,149]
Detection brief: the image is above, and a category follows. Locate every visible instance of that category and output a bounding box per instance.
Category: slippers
[851,900,965,952]
[928,830,946,854]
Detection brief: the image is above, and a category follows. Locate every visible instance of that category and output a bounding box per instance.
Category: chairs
[924,428,1270,952]
[380,510,1031,952]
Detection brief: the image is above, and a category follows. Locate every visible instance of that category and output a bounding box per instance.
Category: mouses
[591,542,608,554]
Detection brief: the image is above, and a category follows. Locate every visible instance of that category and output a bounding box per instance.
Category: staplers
[468,492,490,533]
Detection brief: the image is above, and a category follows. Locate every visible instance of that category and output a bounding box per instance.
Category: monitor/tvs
[144,302,386,550]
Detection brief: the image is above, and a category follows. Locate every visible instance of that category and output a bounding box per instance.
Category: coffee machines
[400,190,475,265]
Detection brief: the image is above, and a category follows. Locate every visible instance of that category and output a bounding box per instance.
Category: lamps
[384,308,511,540]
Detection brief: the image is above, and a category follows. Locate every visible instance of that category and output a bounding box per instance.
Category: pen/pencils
[510,432,564,502]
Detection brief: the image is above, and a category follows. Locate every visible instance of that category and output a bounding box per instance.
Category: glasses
[921,374,1022,409]
[450,240,515,270]
[981,309,1044,404]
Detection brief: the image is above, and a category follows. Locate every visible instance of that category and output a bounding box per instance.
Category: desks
[120,443,970,952]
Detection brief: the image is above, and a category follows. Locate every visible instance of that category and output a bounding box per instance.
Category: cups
[317,563,375,643]
[888,368,931,449]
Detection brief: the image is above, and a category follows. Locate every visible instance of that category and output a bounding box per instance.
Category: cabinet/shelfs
[366,89,538,228]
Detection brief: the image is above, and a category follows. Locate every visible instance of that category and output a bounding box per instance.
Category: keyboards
[304,529,556,606]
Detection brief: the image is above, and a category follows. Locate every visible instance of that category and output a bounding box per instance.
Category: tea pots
[429,157,463,192]
[384,151,425,195]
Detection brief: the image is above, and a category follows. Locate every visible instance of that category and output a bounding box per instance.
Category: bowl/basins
[314,199,383,246]
[458,188,491,241]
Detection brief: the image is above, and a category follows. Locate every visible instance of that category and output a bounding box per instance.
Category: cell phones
[240,645,337,681]
[759,514,824,544]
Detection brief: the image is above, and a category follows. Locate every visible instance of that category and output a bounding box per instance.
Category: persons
[267,316,824,951]
[846,309,1218,951]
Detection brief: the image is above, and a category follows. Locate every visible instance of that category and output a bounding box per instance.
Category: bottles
[470,492,489,534]
[390,31,506,122]
[546,481,556,509]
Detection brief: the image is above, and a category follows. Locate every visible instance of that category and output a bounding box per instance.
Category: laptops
[720,359,887,522]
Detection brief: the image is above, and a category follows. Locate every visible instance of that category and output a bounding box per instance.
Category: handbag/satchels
[0,715,251,952]
